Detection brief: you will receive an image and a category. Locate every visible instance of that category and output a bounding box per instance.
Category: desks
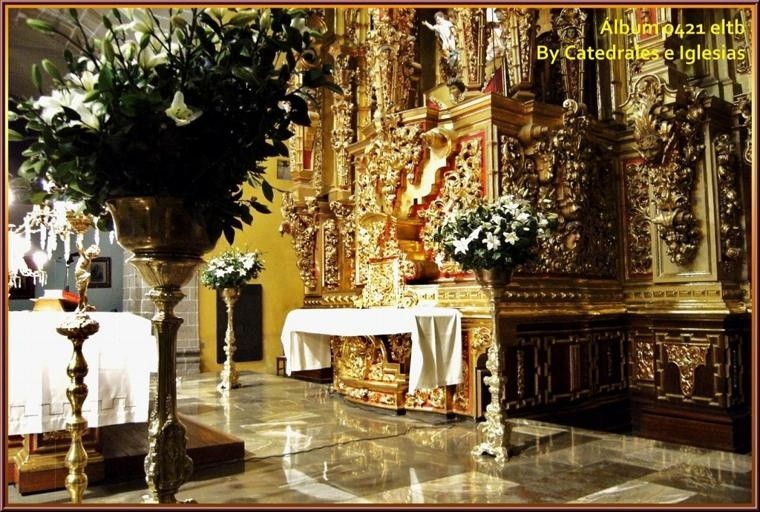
[6,307,154,496]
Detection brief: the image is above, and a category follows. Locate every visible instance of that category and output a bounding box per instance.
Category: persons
[421,11,458,54]
[447,77,466,97]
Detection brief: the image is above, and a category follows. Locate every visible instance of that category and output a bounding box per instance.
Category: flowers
[7,7,345,295]
[422,194,555,281]
